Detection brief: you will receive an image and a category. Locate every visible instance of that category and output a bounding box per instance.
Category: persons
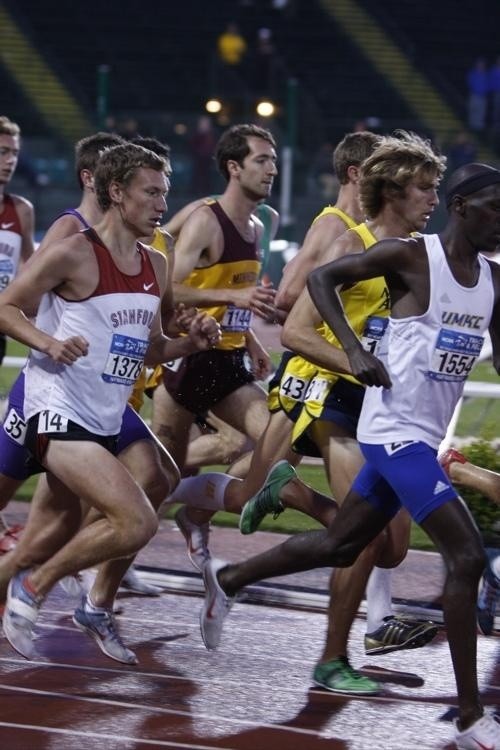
[1,117,500,750]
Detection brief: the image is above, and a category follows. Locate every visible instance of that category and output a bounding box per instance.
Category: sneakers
[4,570,42,661]
[239,459,298,534]
[199,558,239,652]
[58,566,124,613]
[120,566,164,594]
[363,615,437,655]
[73,596,136,663]
[174,507,210,573]
[453,710,500,750]
[438,448,466,479]
[477,564,500,634]
[312,656,383,694]
